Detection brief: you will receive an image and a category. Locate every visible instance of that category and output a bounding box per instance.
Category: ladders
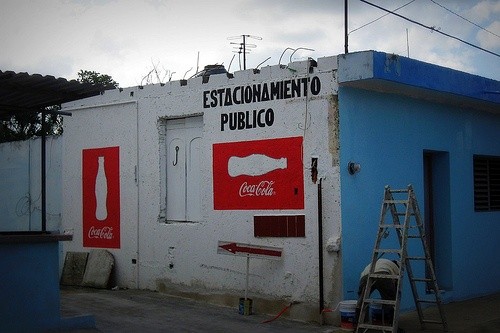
[356,183,449,333]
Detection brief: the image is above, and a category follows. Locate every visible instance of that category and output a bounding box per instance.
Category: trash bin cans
[2,229,74,333]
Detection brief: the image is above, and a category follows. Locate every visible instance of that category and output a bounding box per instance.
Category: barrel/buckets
[338,300,358,331]
[367,298,382,326]
[238,297,253,315]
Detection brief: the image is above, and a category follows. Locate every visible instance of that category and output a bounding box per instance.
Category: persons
[352,259,405,333]
